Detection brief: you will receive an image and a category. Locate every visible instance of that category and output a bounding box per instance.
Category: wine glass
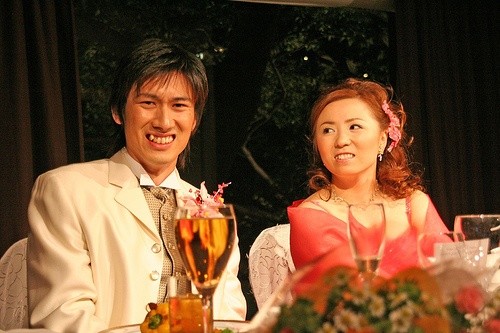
[418,232,467,280]
[347,202,385,284]
[174,203,237,332]
[454,215,500,291]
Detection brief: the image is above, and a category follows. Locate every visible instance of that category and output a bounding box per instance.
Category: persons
[290,78,456,280]
[28,38,246,332]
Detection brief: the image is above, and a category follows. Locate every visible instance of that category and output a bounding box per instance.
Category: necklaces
[324,181,382,208]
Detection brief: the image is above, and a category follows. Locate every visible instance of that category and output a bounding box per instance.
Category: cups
[167,295,215,333]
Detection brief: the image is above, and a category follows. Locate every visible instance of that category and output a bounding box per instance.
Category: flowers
[179,181,232,218]
[267,264,500,333]
[382,100,402,152]
